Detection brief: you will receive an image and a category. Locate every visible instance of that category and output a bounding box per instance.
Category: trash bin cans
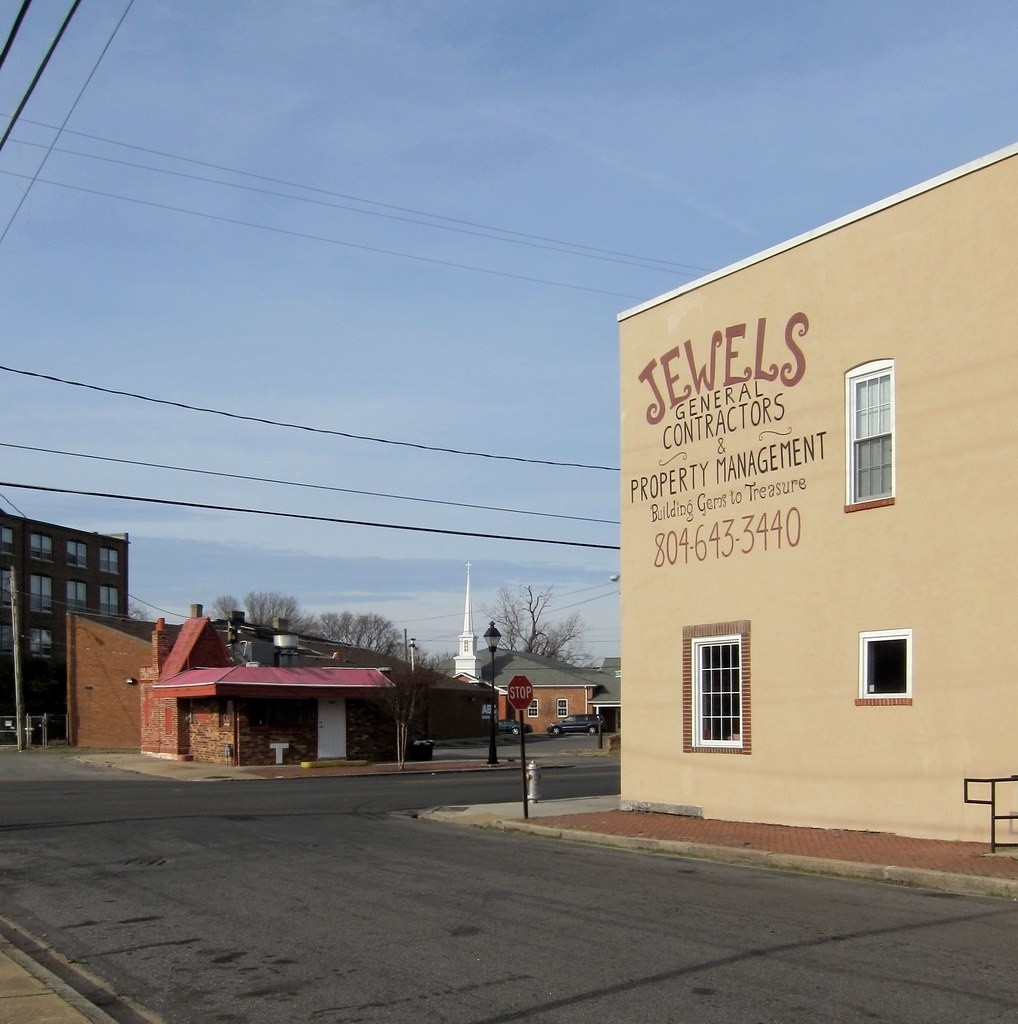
[411,740,434,761]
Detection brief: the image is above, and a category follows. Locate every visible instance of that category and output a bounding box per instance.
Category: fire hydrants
[525,759,541,804]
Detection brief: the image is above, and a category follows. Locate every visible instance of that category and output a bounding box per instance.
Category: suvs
[546,713,606,737]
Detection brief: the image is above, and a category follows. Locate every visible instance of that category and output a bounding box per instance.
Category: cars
[498,719,533,736]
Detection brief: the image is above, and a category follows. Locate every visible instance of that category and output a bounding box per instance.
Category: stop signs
[508,676,533,711]
